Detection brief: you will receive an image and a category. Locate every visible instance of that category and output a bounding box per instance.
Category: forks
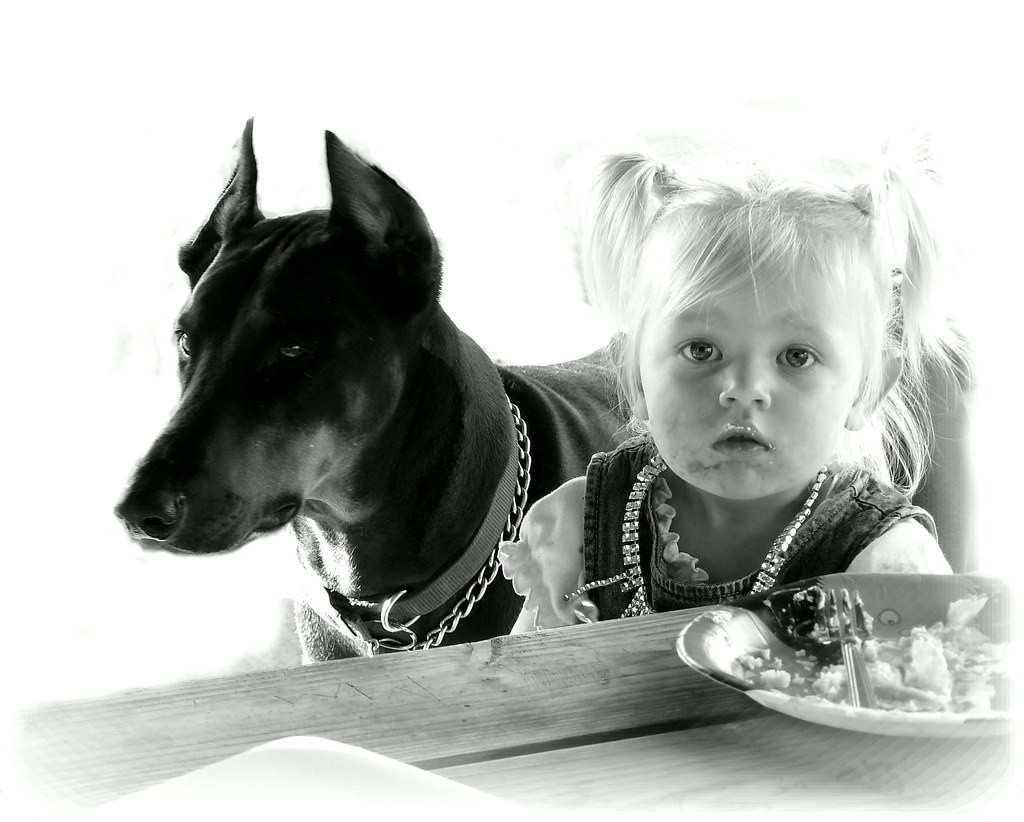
[816,587,877,712]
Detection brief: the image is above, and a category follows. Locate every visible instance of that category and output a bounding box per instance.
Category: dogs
[112,114,979,666]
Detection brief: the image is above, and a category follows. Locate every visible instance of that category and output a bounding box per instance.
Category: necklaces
[562,451,828,623]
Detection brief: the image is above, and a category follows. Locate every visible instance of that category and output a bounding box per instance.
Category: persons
[498,141,953,638]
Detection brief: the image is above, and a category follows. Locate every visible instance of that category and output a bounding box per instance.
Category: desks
[17,599,1012,809]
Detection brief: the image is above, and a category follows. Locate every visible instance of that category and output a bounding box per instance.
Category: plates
[66,736,528,822]
[677,574,1024,736]
